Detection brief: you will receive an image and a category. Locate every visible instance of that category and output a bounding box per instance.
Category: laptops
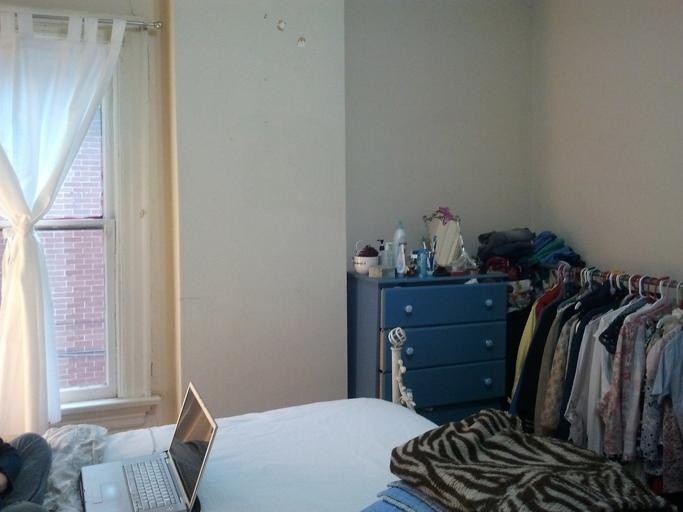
[80,381,218,512]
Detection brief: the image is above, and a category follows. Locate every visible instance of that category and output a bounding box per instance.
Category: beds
[101,326,440,512]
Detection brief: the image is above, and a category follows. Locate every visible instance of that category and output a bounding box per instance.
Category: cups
[353,256,378,275]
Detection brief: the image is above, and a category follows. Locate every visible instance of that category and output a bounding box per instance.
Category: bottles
[378,219,426,277]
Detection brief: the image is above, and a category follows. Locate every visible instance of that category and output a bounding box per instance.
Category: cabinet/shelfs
[348,270,507,426]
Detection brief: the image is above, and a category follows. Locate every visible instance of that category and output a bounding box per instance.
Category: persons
[0,430,51,512]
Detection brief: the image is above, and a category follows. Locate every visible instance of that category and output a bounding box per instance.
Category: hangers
[543,263,683,338]
